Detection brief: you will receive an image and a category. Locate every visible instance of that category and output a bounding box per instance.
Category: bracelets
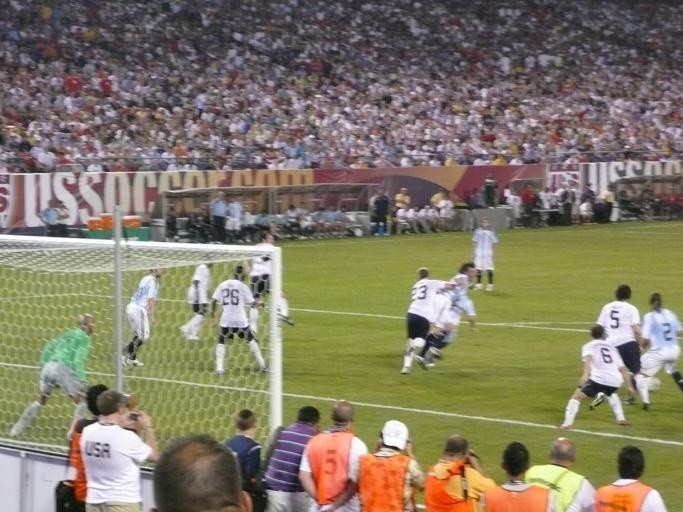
[143,426,153,429]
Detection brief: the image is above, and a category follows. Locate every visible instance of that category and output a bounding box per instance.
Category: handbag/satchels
[55,479,79,512]
[251,478,269,511]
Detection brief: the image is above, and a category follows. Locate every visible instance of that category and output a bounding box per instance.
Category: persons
[523,437,596,512]
[262,406,322,512]
[220,409,261,512]
[9,311,94,441]
[557,325,635,429]
[472,219,498,292]
[414,263,477,371]
[594,445,668,512]
[588,284,642,411]
[69,385,134,512]
[244,234,295,327]
[1,0,683,242]
[179,255,214,342]
[400,267,463,375]
[79,391,160,512]
[633,294,683,410]
[209,266,264,375]
[298,401,368,512]
[482,442,561,512]
[357,419,425,511]
[119,264,162,366]
[424,434,496,512]
[151,434,253,511]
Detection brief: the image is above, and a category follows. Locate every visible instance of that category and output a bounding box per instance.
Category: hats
[381,419,409,451]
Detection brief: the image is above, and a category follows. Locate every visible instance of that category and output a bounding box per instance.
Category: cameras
[129,413,140,421]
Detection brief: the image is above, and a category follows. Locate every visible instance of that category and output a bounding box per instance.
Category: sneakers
[405,337,415,354]
[114,351,127,367]
[279,313,295,326]
[473,285,483,292]
[589,394,607,410]
[127,358,144,367]
[486,285,494,291]
[642,401,651,410]
[622,397,635,405]
[616,418,629,426]
[400,366,411,374]
[261,367,268,374]
[560,424,574,430]
[212,368,225,375]
